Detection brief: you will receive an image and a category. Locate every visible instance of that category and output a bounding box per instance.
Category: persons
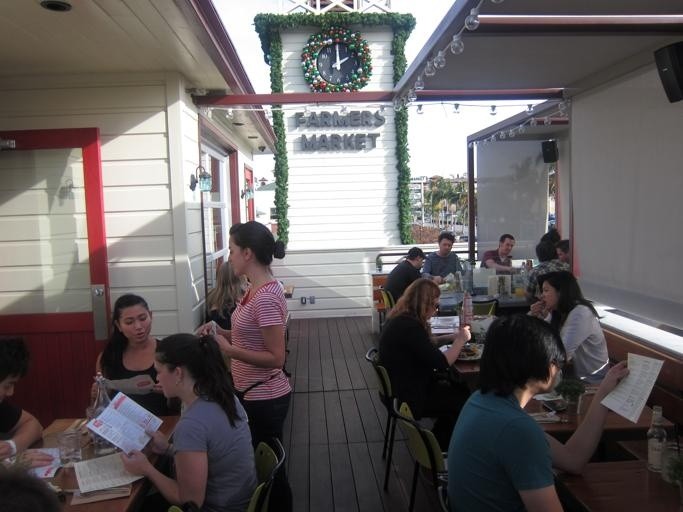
[386,247,424,304]
[95,294,180,416]
[422,232,462,283]
[524,232,569,300]
[205,261,250,332]
[447,316,629,512]
[214,221,294,512]
[480,234,515,276]
[120,333,257,512]
[0,332,54,470]
[531,271,610,386]
[379,279,472,425]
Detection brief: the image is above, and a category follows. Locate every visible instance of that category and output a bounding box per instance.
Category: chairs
[378,285,396,313]
[455,298,499,316]
[167,429,285,512]
[377,390,449,512]
[371,273,390,333]
[364,347,441,491]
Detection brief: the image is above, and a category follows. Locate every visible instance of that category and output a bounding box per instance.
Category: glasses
[432,303,440,309]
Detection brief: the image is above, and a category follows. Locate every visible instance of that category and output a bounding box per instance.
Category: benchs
[602,328,683,460]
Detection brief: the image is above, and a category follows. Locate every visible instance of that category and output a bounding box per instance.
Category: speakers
[540,139,560,165]
[651,40,683,105]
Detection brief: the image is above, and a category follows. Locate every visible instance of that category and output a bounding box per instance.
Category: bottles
[462,291,474,324]
[90,374,119,455]
[647,406,667,473]
[520,262,526,273]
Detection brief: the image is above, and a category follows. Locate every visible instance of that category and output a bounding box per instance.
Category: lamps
[378,106,387,117]
[450,35,464,55]
[433,51,446,68]
[482,139,489,147]
[558,102,565,110]
[340,105,349,115]
[526,104,534,115]
[264,108,272,120]
[509,130,515,138]
[453,104,461,116]
[225,108,233,119]
[190,165,213,193]
[491,134,497,143]
[425,61,436,76]
[544,116,552,127]
[500,131,506,139]
[518,124,525,134]
[416,104,423,115]
[393,99,402,112]
[465,7,480,31]
[241,188,251,200]
[407,88,418,102]
[303,106,311,118]
[489,105,497,116]
[530,117,537,127]
[414,75,425,90]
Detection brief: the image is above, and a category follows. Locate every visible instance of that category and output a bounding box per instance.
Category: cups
[87,409,105,437]
[57,431,80,468]
[662,442,683,483]
[93,429,114,455]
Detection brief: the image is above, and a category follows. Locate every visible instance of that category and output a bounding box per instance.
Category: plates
[443,348,483,360]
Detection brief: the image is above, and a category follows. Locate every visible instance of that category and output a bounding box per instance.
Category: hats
[408,247,429,260]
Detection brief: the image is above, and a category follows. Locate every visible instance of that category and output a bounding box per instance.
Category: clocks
[301,24,373,93]
